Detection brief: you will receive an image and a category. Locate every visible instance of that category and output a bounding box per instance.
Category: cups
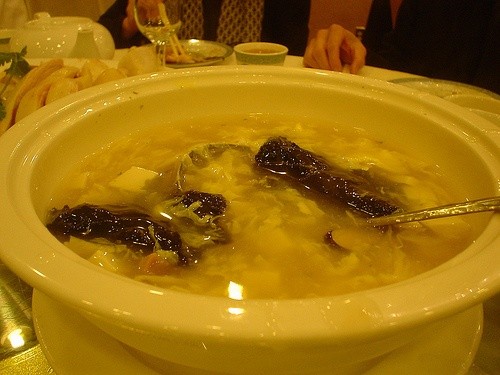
[234,42,288,66]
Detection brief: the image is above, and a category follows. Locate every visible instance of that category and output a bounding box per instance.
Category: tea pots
[8,12,115,60]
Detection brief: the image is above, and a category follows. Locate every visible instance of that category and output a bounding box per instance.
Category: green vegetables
[0,45,34,120]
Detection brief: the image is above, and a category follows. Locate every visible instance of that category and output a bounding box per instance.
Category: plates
[167,39,233,68]
[32,287,484,375]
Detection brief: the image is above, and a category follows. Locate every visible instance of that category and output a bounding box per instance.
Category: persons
[96,0,311,57]
[303,0,500,98]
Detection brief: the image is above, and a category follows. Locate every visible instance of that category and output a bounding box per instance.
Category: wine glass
[133,0,184,72]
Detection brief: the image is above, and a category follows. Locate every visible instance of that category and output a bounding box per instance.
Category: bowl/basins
[0,65,500,375]
